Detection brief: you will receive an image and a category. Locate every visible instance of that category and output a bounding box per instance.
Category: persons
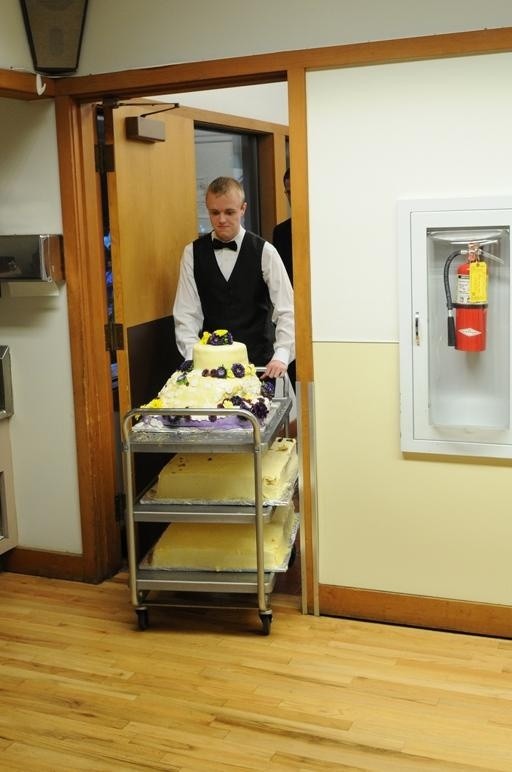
[173,175,296,442]
[273,167,293,290]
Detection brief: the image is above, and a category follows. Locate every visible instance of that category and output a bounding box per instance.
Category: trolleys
[118,359,298,638]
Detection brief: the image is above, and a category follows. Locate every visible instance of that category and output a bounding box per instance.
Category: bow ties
[211,237,237,253]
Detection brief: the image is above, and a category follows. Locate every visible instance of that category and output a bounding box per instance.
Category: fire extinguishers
[443,241,487,352]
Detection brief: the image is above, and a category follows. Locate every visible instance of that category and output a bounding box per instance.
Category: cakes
[157,437,300,503]
[151,500,296,569]
[159,329,275,423]
